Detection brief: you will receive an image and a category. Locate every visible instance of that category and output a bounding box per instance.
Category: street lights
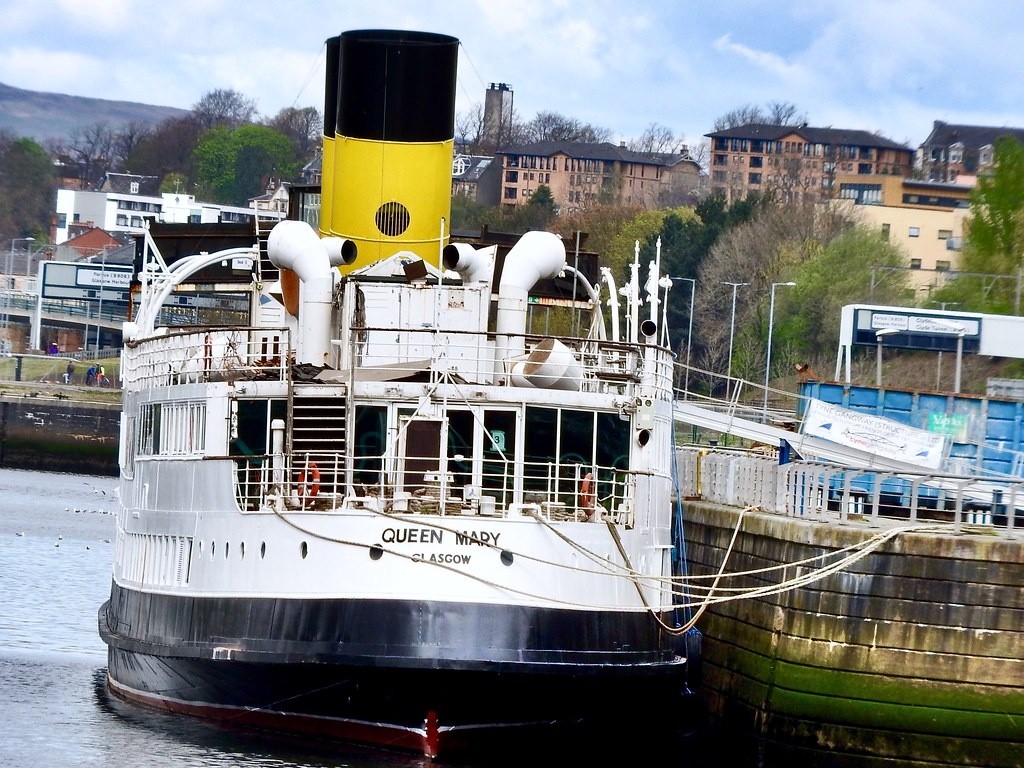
[3,237,36,353]
[762,281,797,422]
[719,281,752,402]
[669,276,695,402]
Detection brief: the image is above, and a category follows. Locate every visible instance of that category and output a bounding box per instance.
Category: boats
[98,28,704,758]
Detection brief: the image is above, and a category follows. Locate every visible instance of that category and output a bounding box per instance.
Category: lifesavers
[579,472,597,517]
[297,460,321,506]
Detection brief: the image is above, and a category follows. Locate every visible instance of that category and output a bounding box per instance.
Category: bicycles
[91,374,110,389]
